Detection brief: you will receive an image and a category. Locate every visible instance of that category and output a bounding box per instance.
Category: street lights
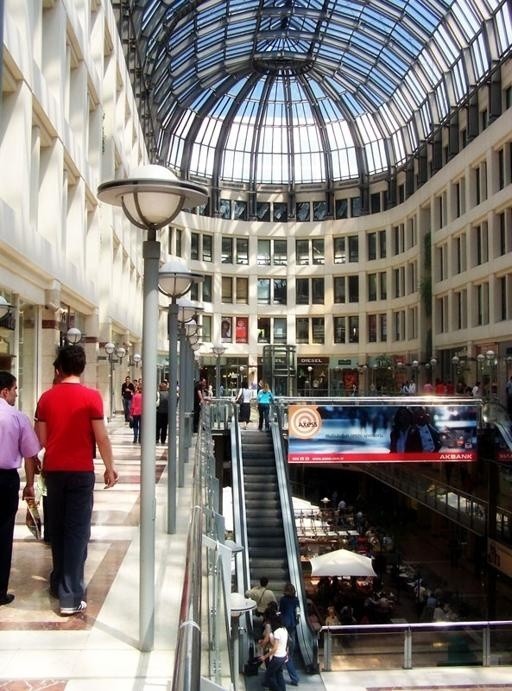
[425,358,438,386]
[92,161,212,652]
[224,539,245,559]
[66,327,82,346]
[387,362,405,379]
[0,293,17,323]
[452,356,465,395]
[229,592,257,691]
[210,342,228,399]
[307,366,313,395]
[156,255,206,537]
[476,350,499,400]
[133,352,141,369]
[362,364,378,391]
[412,360,421,393]
[159,294,205,489]
[104,343,126,422]
[239,366,245,388]
[156,362,164,385]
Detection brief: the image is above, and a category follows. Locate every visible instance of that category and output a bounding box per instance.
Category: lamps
[0,297,17,321]
[58,328,88,344]
[450,348,499,371]
[105,343,142,370]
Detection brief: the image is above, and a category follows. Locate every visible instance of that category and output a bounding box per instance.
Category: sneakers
[60,601,87,615]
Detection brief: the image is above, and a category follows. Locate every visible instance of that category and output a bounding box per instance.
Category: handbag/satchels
[236,393,244,404]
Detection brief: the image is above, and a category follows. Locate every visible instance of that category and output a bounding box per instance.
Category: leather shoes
[0,595,14,605]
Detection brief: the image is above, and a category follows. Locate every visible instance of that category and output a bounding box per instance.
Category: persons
[245,580,301,691]
[299,368,512,417]
[1,369,39,605]
[122,363,229,446]
[21,360,121,545]
[37,343,118,618]
[309,489,509,645]
[256,383,275,432]
[235,381,252,430]
